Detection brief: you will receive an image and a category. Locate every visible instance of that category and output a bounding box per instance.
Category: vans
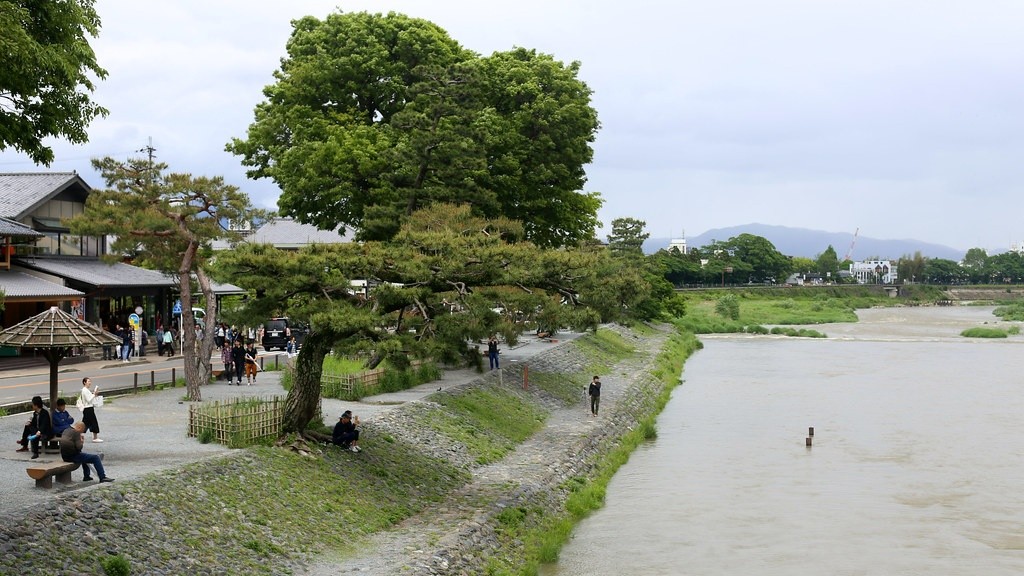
[262,317,292,351]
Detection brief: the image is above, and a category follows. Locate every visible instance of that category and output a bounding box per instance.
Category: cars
[191,307,207,321]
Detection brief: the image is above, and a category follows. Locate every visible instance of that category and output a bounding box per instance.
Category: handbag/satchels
[92,393,103,407]
[76,396,85,412]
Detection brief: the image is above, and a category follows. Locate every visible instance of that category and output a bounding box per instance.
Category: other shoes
[100,477,115,483]
[17,441,21,444]
[248,383,251,385]
[349,446,358,452]
[171,352,175,356]
[31,454,39,459]
[594,415,598,417]
[92,438,103,443]
[123,360,129,363]
[253,380,256,384]
[236,381,240,385]
[228,381,231,385]
[16,447,28,452]
[355,446,361,451]
[83,477,93,481]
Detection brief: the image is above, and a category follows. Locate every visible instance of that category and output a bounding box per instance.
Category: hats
[342,413,351,418]
[57,399,66,406]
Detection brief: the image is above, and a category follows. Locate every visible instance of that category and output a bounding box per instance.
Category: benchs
[212,369,236,380]
[38,438,62,452]
[27,460,80,489]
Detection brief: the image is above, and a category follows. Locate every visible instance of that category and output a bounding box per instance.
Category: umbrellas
[0,306,123,452]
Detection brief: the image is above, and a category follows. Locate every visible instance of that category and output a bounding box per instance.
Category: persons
[101,321,256,363]
[60,421,115,483]
[487,335,501,370]
[15,396,53,459]
[221,340,234,385]
[333,410,361,453]
[231,339,246,385]
[244,341,258,386]
[287,335,301,358]
[79,377,104,443]
[589,376,601,417]
[53,398,75,438]
